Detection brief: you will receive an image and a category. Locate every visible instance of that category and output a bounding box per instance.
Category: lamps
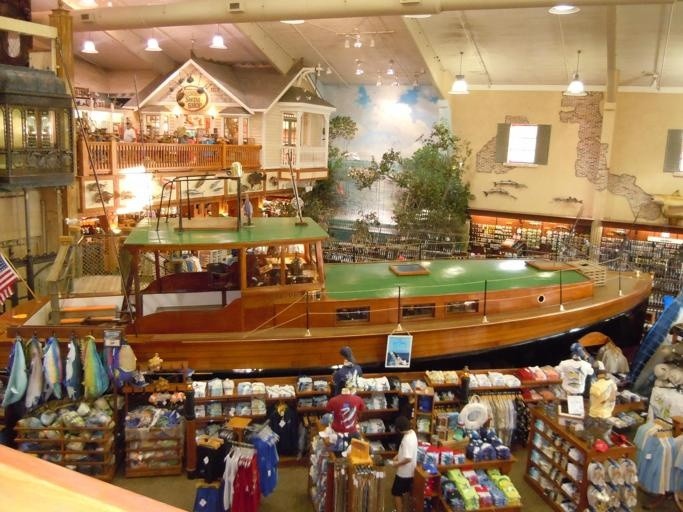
[562,50,588,96]
[447,52,468,94]
[143,28,163,51]
[80,31,100,53]
[207,23,228,49]
[548,4,580,14]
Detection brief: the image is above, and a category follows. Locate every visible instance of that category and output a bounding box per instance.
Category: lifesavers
[457,404,488,431]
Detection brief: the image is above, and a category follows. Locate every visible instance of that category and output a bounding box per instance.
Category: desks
[120,216,329,319]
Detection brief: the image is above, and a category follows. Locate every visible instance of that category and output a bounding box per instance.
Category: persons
[319,386,370,444]
[585,368,619,447]
[554,342,595,395]
[124,121,137,142]
[387,417,418,512]
[331,347,363,397]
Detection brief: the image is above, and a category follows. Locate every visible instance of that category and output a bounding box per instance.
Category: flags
[0,254,20,306]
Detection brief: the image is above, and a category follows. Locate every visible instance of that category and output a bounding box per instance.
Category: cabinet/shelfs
[464,207,683,326]
[12,360,645,512]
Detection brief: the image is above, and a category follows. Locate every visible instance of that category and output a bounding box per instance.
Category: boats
[1,215,654,380]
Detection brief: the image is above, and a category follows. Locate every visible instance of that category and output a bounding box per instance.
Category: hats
[570,343,583,354]
[594,360,604,369]
